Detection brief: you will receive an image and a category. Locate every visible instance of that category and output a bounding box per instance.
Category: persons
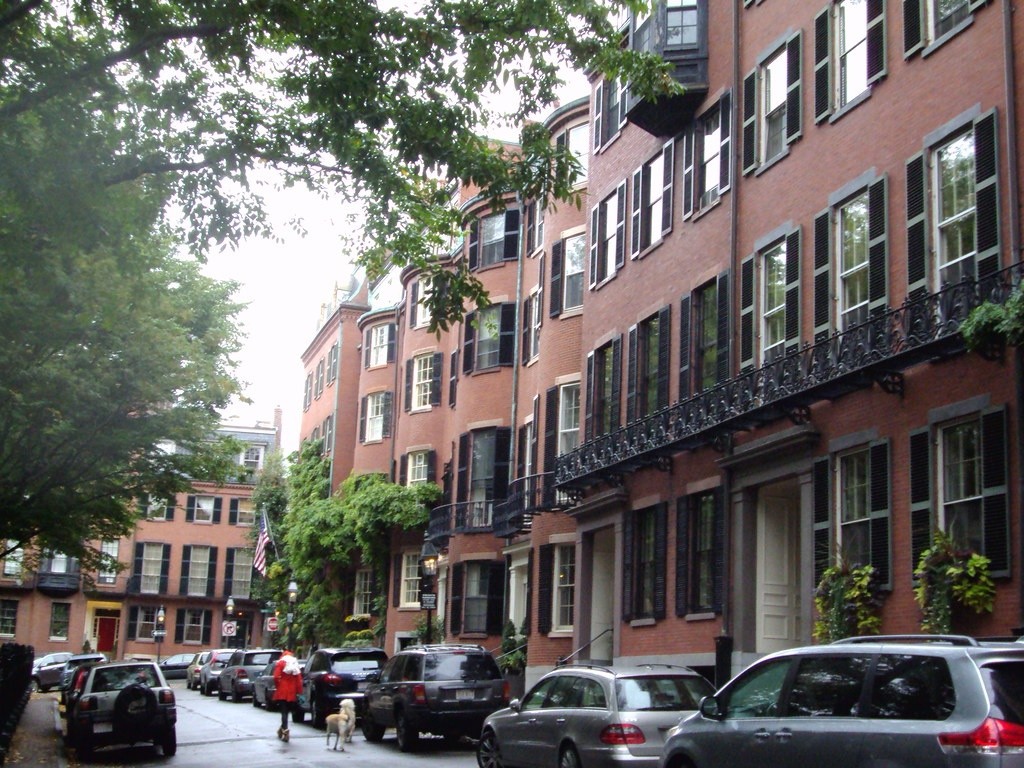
[270,650,303,742]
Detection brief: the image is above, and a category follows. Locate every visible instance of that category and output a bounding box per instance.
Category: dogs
[325,713,349,751]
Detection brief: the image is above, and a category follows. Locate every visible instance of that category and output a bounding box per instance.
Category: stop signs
[267,616,280,632]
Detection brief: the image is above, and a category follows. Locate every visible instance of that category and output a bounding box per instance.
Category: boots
[278,728,290,742]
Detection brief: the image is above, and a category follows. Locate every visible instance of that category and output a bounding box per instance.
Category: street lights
[419,529,439,644]
[223,595,235,648]
[154,605,165,662]
[284,571,299,649]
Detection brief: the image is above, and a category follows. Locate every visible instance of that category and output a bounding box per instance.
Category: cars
[65,663,109,744]
[248,659,312,710]
[186,652,212,692]
[156,654,197,679]
[25,652,110,694]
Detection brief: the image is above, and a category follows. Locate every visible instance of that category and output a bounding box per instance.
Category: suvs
[361,643,509,755]
[218,648,290,703]
[58,663,177,765]
[200,649,242,697]
[660,632,1024,768]
[286,647,394,731]
[473,664,720,767]
